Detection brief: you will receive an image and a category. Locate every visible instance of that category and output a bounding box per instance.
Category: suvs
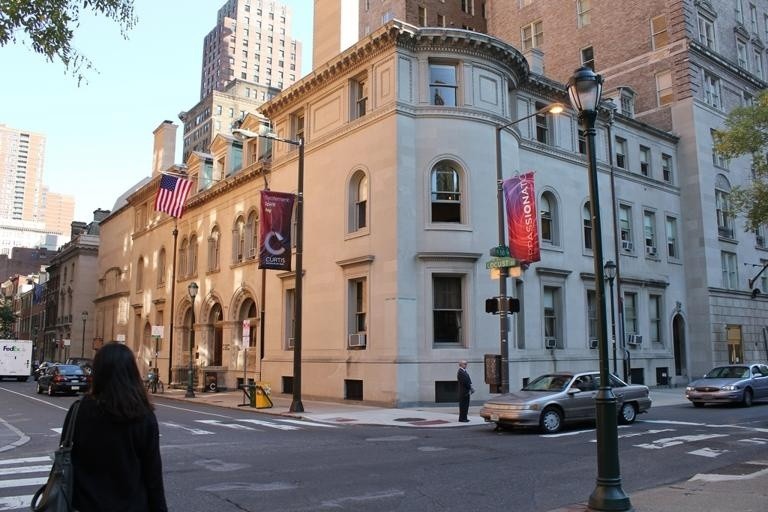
[65,357,94,368]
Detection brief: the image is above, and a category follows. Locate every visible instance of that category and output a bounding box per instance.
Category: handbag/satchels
[31,450,74,511]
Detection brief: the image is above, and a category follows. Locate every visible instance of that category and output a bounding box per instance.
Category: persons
[30,340,168,512]
[457,360,477,422]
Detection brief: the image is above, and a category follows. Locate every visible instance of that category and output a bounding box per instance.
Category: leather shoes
[459,420,469,422]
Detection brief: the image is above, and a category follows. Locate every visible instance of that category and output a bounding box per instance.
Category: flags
[154,173,194,219]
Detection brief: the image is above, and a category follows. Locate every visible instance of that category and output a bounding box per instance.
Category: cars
[479,370,653,434]
[35,363,88,396]
[35,361,63,380]
[686,363,768,407]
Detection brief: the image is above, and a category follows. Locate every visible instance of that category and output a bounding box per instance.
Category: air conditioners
[347,333,366,347]
[646,246,656,255]
[544,337,557,349]
[589,339,600,349]
[627,334,642,345]
[288,337,294,349]
[622,240,632,251]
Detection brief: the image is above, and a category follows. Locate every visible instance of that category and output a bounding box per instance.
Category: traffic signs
[486,259,517,269]
[493,247,511,257]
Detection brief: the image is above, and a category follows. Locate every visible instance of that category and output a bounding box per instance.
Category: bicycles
[145,374,164,394]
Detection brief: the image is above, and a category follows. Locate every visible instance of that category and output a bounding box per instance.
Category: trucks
[0,338,36,382]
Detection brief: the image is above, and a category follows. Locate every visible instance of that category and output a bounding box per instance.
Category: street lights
[604,261,619,375]
[185,281,199,398]
[488,104,563,395]
[169,227,179,388]
[565,63,634,512]
[233,128,306,413]
[82,309,88,359]
